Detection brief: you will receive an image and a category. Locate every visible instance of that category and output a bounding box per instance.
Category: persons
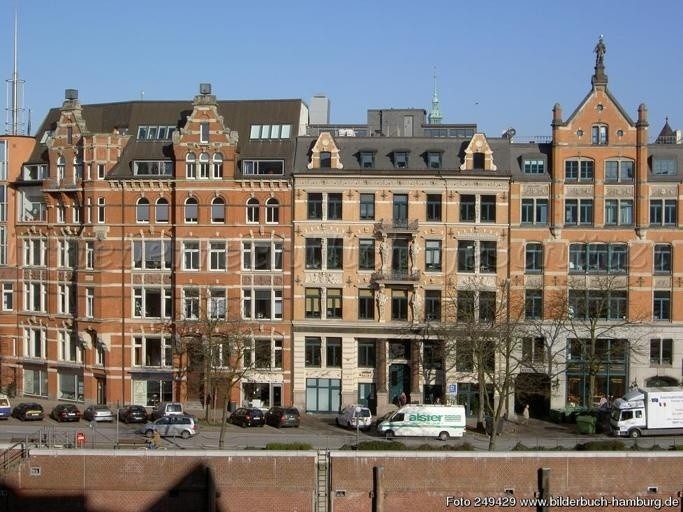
[521,403,530,426]
[397,392,407,405]
[152,429,161,449]
[433,397,442,404]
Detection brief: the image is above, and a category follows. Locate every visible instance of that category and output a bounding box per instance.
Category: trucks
[609,386,683,438]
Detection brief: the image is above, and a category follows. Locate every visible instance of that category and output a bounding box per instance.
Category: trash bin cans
[75,431,84,448]
[576,416,596,435]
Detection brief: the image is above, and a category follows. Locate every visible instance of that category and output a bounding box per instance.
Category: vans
[140,415,196,439]
[0,393,11,420]
[377,403,466,441]
[264,406,299,428]
[335,404,372,429]
[228,407,265,428]
[151,402,183,419]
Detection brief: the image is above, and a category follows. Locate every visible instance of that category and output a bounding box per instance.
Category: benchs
[117,438,151,449]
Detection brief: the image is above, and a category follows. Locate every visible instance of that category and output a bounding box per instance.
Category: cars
[84,404,114,422]
[51,404,81,421]
[119,404,148,424]
[13,403,44,421]
